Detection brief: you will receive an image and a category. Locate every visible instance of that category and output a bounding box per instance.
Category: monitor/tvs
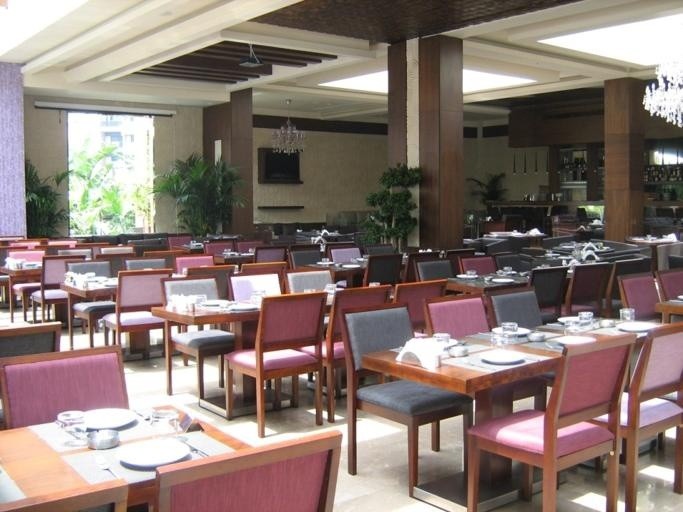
[258,147,304,185]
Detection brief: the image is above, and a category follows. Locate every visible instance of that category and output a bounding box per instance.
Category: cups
[434,332,450,356]
[501,322,519,345]
[325,284,336,304]
[321,258,329,268]
[620,308,634,320]
[577,312,595,333]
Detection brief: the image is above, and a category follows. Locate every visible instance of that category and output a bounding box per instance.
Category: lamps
[269,101,310,156]
[639,37,683,131]
[510,151,599,174]
[644,147,682,181]
[237,44,266,70]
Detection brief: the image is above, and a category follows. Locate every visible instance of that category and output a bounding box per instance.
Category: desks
[2,404,255,512]
[359,320,681,512]
[152,287,334,421]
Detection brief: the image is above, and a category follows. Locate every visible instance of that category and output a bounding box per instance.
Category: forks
[444,341,466,350]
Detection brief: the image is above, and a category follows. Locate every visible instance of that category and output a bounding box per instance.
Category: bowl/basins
[86,430,119,449]
[449,346,468,357]
[599,319,615,328]
[526,333,545,342]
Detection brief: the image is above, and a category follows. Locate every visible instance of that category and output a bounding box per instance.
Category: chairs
[30,253,87,324]
[582,321,683,512]
[0,478,132,512]
[481,287,555,393]
[151,429,345,512]
[1,345,132,430]
[0,320,63,357]
[222,290,330,439]
[617,272,661,322]
[340,302,475,498]
[465,331,639,512]
[158,275,234,400]
[63,259,119,351]
[420,293,548,453]
[653,266,683,301]
[226,270,283,304]
[0,224,682,322]
[282,268,335,297]
[100,268,174,362]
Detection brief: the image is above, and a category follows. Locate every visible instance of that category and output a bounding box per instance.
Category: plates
[618,321,658,333]
[315,261,334,265]
[558,316,578,325]
[342,264,360,269]
[480,351,524,366]
[662,193,671,201]
[493,325,530,335]
[87,277,116,286]
[80,407,135,432]
[556,334,596,346]
[456,266,522,283]
[116,437,189,470]
[57,410,81,423]
[201,298,257,313]
